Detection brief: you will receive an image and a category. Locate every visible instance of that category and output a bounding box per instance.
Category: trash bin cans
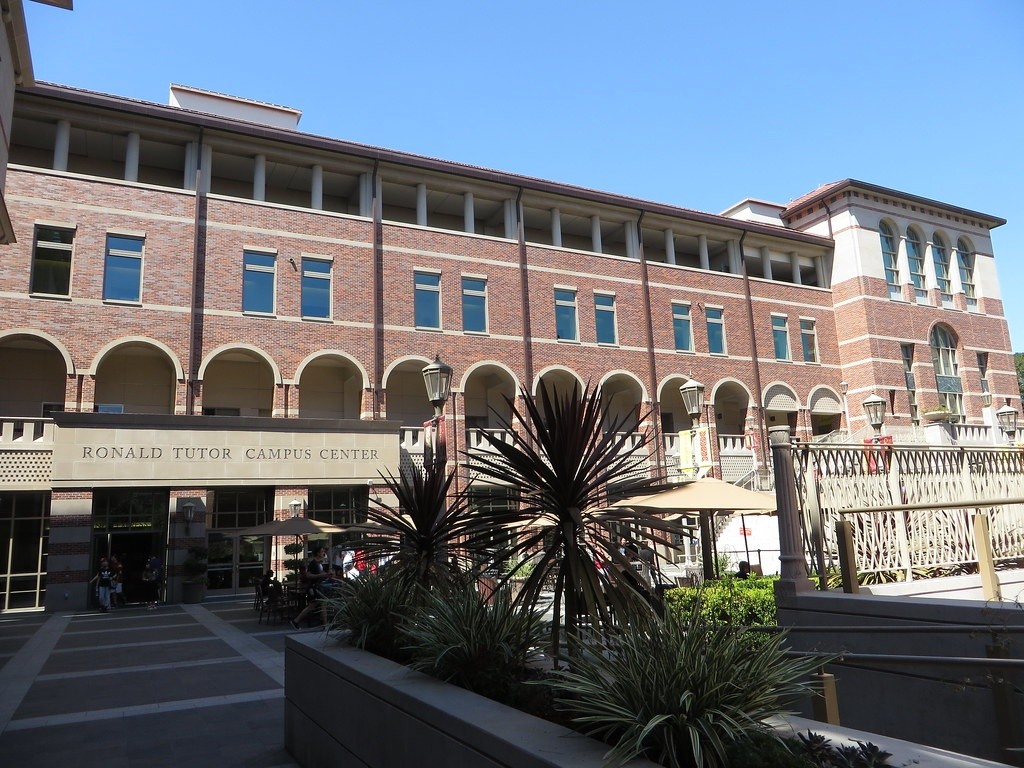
[509,577,532,605]
[477,570,500,606]
[608,561,652,596]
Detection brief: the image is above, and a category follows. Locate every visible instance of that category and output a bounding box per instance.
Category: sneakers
[111,604,118,609]
[322,625,333,631]
[147,604,158,611]
[291,619,302,631]
[99,605,107,613]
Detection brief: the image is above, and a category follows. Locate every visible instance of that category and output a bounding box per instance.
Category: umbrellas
[252,516,349,605]
[347,520,397,574]
[610,477,776,577]
[225,520,283,580]
[360,514,460,533]
[500,506,639,529]
[731,494,802,572]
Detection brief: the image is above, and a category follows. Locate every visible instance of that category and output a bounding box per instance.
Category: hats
[100,557,106,562]
[266,570,273,573]
[452,559,459,564]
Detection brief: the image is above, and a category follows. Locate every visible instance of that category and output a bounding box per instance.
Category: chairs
[253,580,319,626]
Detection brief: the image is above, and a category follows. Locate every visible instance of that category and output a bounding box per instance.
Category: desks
[284,583,311,619]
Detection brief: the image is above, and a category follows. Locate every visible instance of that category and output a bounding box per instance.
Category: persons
[261,570,277,605]
[330,541,376,579]
[734,561,750,579]
[143,554,164,606]
[89,555,123,613]
[587,532,658,592]
[290,546,333,630]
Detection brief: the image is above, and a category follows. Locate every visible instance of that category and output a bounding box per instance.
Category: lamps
[839,380,849,395]
[745,415,755,431]
[183,497,196,536]
[289,497,302,519]
[980,388,992,407]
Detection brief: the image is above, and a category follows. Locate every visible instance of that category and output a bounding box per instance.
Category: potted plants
[284,379,1024,768]
[921,404,951,421]
